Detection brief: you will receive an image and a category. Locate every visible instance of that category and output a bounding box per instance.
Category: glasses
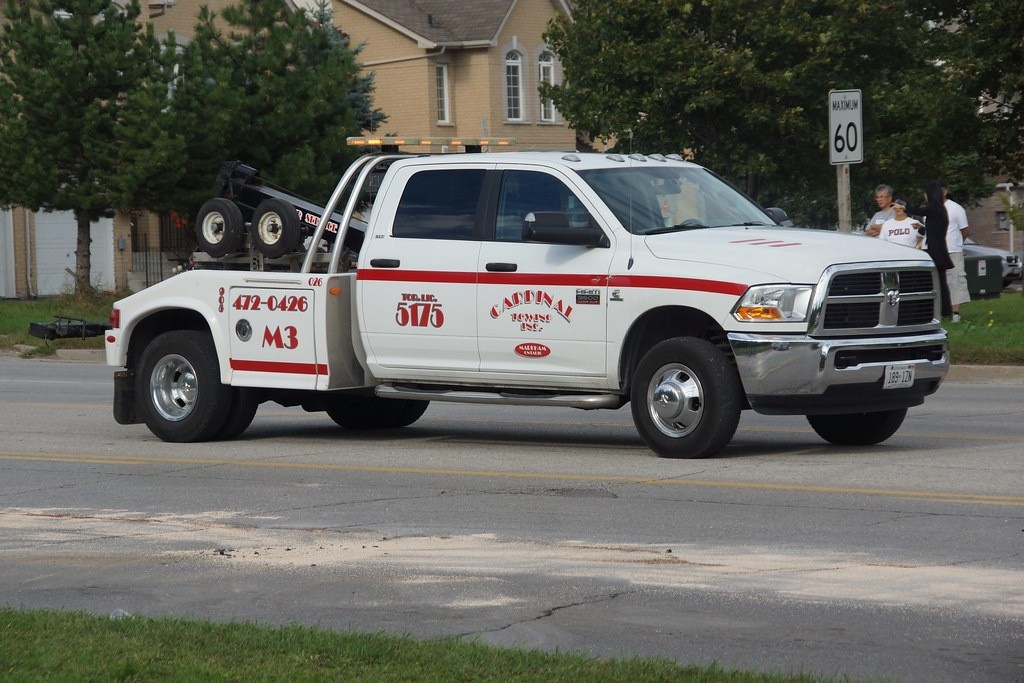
[875,194,889,200]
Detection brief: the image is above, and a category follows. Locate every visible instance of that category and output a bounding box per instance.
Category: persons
[939,182,972,322]
[866,183,955,321]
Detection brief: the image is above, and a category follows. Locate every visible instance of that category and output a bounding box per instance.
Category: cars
[958,236,1023,291]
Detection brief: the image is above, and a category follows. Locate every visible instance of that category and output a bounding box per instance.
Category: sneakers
[952,315,961,323]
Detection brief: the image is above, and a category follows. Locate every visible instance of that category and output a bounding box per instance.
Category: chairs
[502,177,594,234]
[441,176,480,234]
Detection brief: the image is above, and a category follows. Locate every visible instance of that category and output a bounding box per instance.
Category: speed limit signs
[828,88,864,166]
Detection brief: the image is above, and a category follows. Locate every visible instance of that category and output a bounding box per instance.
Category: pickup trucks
[103,133,952,458]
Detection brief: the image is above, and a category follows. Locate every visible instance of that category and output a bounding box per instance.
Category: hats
[890,199,906,208]
